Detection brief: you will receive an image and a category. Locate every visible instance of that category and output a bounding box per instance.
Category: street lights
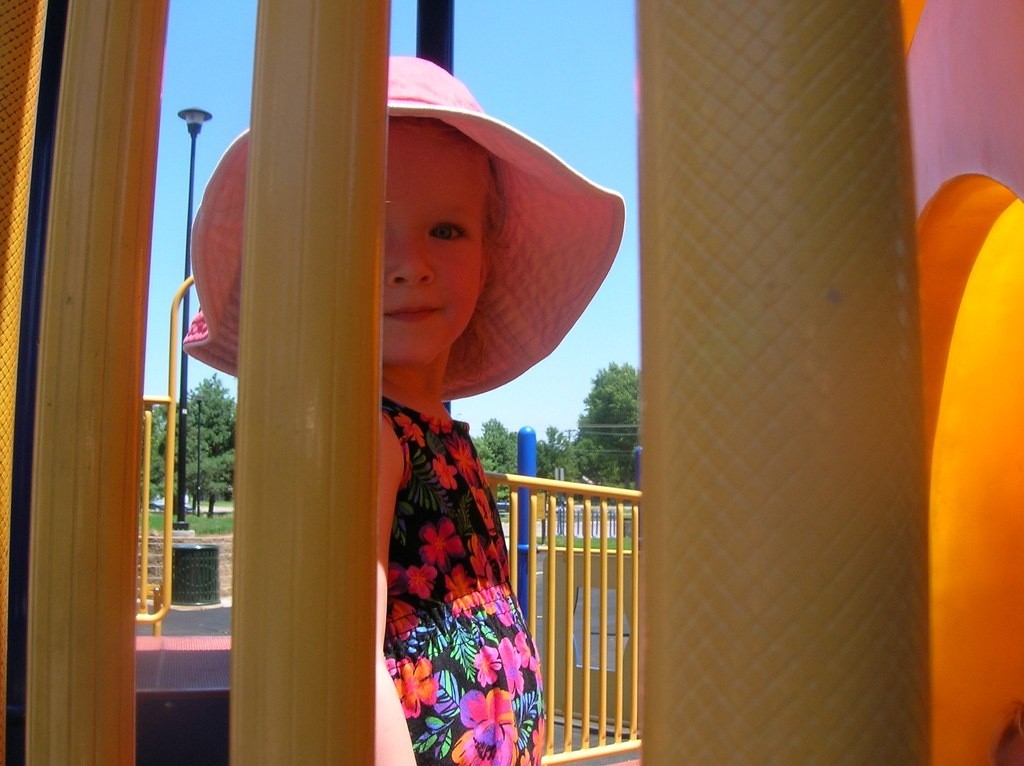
[173,107,213,530]
[195,393,205,518]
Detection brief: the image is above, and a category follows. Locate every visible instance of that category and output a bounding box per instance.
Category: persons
[190,56,626,766]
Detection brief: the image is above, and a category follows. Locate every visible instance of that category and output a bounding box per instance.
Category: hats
[181,54,627,403]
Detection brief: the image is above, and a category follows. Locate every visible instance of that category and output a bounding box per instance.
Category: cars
[148,499,194,512]
[496,503,510,510]
